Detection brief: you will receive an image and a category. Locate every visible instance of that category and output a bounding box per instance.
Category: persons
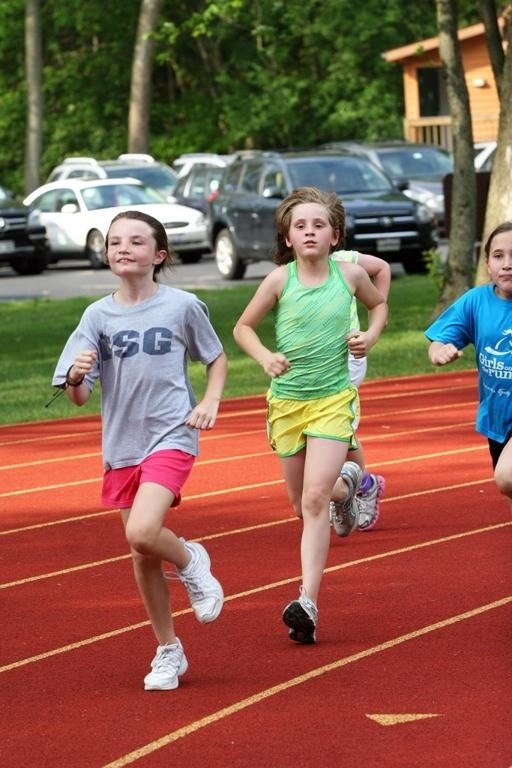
[276,236,392,535]
[424,222,512,498]
[50,210,228,692]
[233,186,386,647]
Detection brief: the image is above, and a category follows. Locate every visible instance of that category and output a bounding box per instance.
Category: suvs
[204,144,441,281]
[42,151,180,204]
[311,139,454,242]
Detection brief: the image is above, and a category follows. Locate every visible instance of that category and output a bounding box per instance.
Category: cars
[21,175,213,270]
[171,148,262,212]
[0,183,50,276]
[473,141,498,173]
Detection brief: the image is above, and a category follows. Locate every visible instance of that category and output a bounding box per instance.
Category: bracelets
[64,365,86,388]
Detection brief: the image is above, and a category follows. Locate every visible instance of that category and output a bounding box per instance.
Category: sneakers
[143,635,188,692]
[282,596,317,644]
[331,462,363,537]
[355,473,386,531]
[174,536,224,625]
